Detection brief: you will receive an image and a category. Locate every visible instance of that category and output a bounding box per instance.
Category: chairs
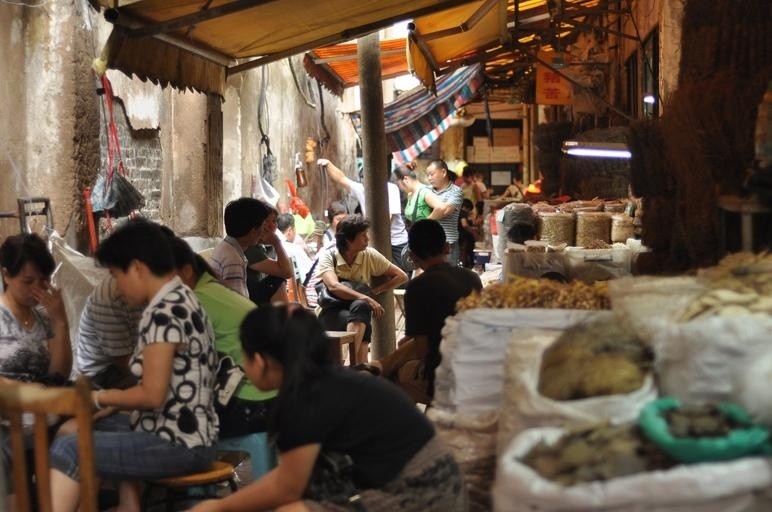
[0,373,96,511]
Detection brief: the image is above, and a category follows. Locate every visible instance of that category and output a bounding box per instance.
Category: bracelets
[93,388,108,411]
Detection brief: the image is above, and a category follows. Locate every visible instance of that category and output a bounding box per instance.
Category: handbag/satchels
[400,243,419,270]
[315,276,375,310]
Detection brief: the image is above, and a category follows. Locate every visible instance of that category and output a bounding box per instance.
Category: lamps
[561,140,632,159]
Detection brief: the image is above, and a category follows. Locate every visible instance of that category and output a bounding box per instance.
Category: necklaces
[3,298,32,328]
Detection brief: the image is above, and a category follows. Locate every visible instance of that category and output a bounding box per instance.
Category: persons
[2,234,73,509]
[49,217,219,510]
[301,153,542,401]
[72,197,311,402]
[172,235,280,442]
[184,302,468,510]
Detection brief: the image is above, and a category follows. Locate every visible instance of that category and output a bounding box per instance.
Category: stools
[392,288,407,339]
[140,459,243,511]
[323,328,358,366]
